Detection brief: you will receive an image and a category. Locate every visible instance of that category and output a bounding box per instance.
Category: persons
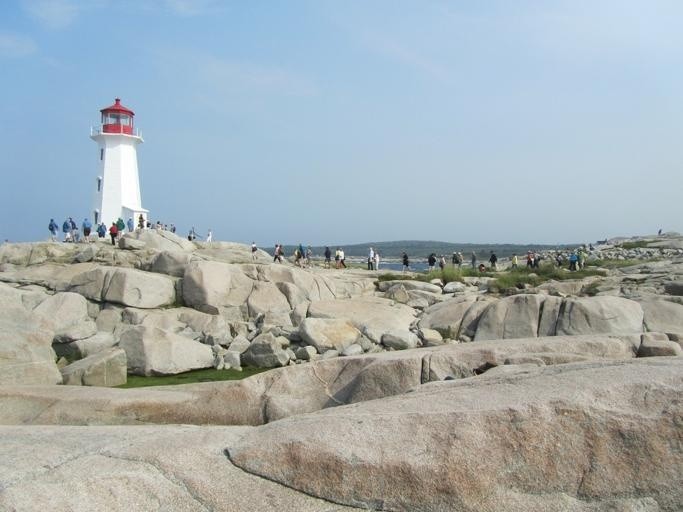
[47,213,214,246]
[368,248,411,271]
[511,243,595,272]
[427,250,497,274]
[251,241,348,269]
